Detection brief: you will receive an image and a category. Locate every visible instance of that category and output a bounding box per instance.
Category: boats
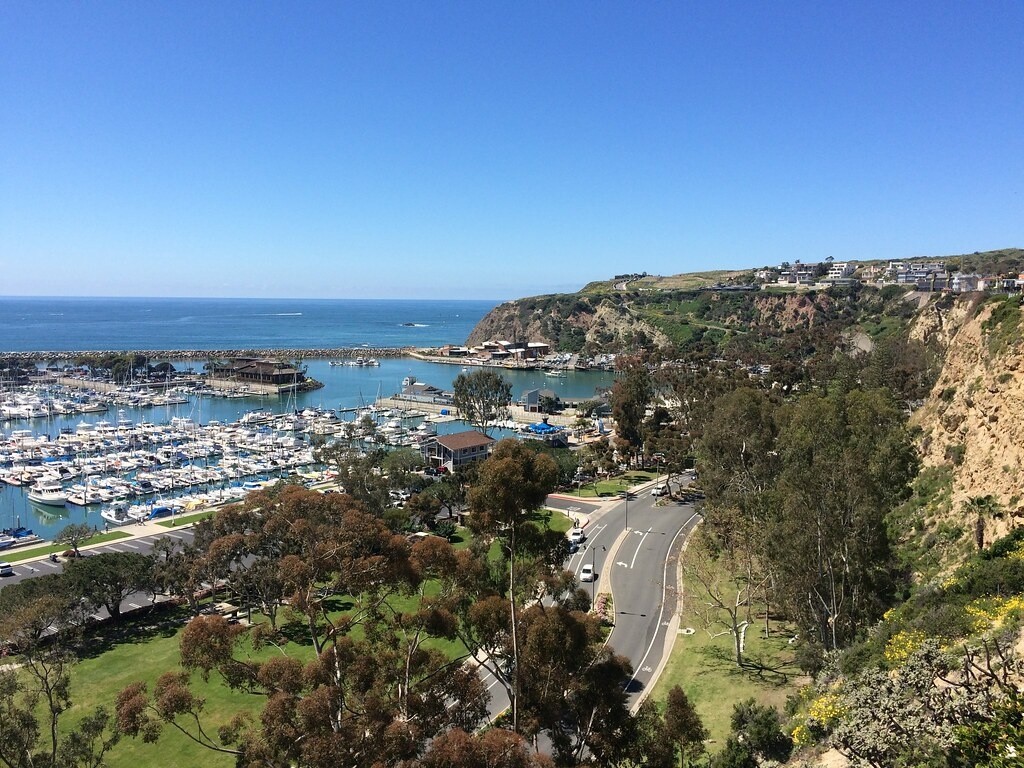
[544,368,567,378]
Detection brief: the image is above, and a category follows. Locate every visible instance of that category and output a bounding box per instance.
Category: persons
[574,518,580,529]
[133,515,145,526]
[105,520,108,533]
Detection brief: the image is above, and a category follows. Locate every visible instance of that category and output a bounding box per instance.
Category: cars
[571,528,585,543]
[388,488,411,501]
[651,484,667,496]
[580,564,593,582]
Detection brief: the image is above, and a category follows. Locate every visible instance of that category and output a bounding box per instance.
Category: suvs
[423,468,439,476]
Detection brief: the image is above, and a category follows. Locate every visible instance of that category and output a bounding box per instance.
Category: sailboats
[0,356,533,527]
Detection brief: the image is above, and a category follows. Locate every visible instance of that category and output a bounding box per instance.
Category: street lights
[583,544,606,612]
[621,481,636,532]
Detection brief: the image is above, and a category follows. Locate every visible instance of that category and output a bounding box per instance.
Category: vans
[437,466,447,472]
